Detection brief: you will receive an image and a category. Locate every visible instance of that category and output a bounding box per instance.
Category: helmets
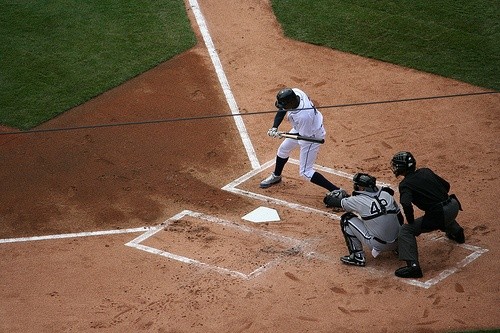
[353,173,376,188]
[275,88,297,110]
[390,151,416,175]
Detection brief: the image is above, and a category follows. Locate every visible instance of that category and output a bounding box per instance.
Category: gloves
[268,128,277,138]
[275,131,286,140]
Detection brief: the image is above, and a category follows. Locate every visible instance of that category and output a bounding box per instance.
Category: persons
[260,87,343,192]
[323,172,404,266]
[389,151,465,278]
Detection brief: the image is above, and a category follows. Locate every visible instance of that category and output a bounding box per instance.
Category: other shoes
[395,265,423,278]
[445,233,465,244]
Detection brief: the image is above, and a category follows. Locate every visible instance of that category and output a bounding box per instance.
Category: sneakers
[328,188,347,199]
[260,172,281,188]
[340,251,366,266]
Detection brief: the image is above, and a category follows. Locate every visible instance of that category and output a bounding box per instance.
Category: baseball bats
[280,133,324,144]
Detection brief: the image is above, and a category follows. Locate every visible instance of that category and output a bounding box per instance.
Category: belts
[373,237,398,244]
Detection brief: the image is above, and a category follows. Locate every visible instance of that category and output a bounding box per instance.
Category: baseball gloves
[322,189,348,208]
